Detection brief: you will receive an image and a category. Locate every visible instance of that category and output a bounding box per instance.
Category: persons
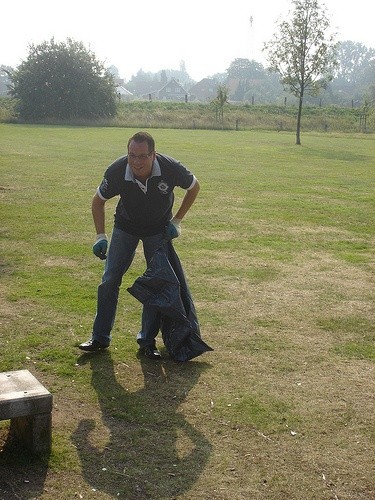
[79,131,201,358]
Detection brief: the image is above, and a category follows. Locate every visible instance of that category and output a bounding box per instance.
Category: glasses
[128,153,152,160]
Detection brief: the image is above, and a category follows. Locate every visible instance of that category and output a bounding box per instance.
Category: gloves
[164,216,182,241]
[92,232,108,260]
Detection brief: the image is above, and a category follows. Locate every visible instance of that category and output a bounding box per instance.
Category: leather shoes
[144,347,161,360]
[79,338,109,351]
